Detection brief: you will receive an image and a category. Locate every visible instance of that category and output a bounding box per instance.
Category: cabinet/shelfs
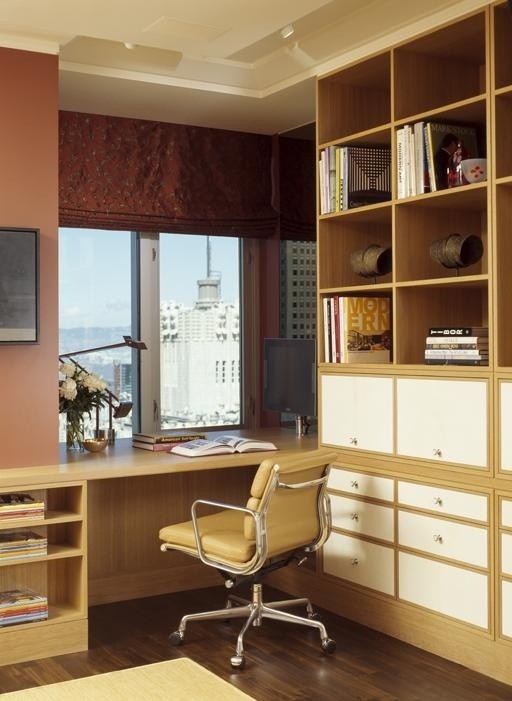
[492,1,512,688]
[0,481,88,667]
[286,4,495,677]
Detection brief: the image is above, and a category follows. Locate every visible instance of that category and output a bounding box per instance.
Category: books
[318,146,392,214]
[424,327,488,365]
[166,435,280,458]
[397,122,479,198]
[0,587,48,625]
[131,430,206,451]
[0,531,48,560]
[0,493,44,522]
[323,296,391,364]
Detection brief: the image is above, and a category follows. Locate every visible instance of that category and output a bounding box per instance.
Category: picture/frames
[0,224,41,345]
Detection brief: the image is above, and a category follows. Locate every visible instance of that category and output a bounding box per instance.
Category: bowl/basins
[460,158,487,183]
[82,438,109,453]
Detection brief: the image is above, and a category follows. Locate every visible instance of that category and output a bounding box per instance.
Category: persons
[433,136,466,192]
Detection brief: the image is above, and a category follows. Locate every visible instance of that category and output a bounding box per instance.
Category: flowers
[58,362,108,446]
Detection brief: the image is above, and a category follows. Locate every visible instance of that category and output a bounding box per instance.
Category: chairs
[157,450,339,670]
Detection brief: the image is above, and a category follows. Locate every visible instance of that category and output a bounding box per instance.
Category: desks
[0,422,330,488]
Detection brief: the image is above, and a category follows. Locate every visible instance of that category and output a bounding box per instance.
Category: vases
[66,409,86,452]
